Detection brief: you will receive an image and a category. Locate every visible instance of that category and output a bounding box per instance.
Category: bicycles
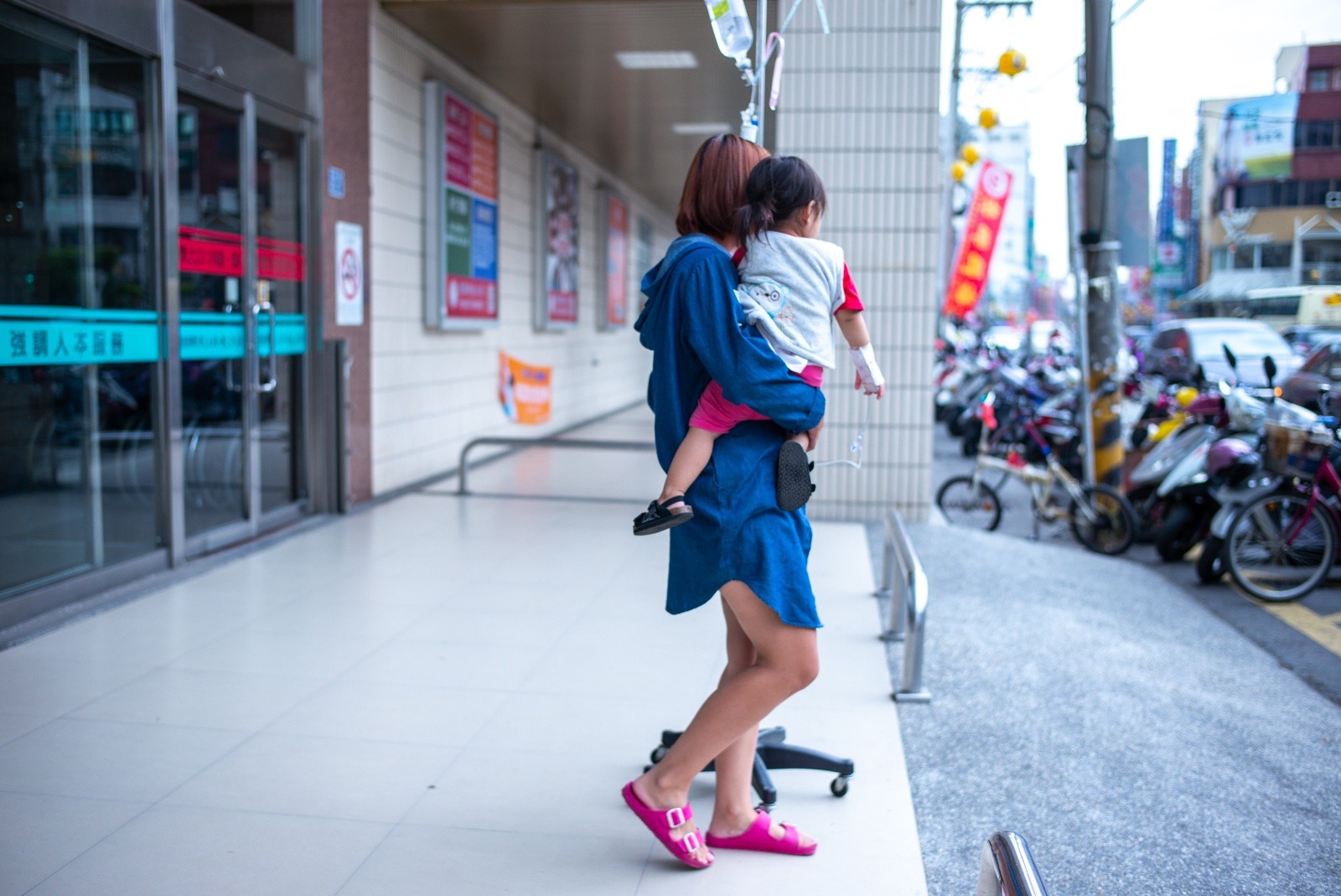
[1222,414,1341,603]
[936,392,1136,557]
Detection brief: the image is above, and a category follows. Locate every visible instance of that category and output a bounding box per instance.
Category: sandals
[778,438,816,511]
[633,496,693,535]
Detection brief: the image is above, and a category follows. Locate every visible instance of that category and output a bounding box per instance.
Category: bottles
[703,0,754,70]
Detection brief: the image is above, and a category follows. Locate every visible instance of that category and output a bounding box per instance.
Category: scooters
[929,331,1341,586]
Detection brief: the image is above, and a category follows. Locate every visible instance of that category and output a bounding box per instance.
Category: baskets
[1264,421,1331,481]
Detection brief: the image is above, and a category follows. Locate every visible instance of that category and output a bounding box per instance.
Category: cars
[1282,324,1341,377]
[978,318,1079,358]
[1133,316,1307,405]
[1279,336,1341,425]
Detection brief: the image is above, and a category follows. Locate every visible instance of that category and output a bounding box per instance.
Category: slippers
[706,809,817,855]
[622,782,716,869]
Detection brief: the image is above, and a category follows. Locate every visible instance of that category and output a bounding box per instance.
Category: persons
[629,156,884,534]
[621,134,824,870]
